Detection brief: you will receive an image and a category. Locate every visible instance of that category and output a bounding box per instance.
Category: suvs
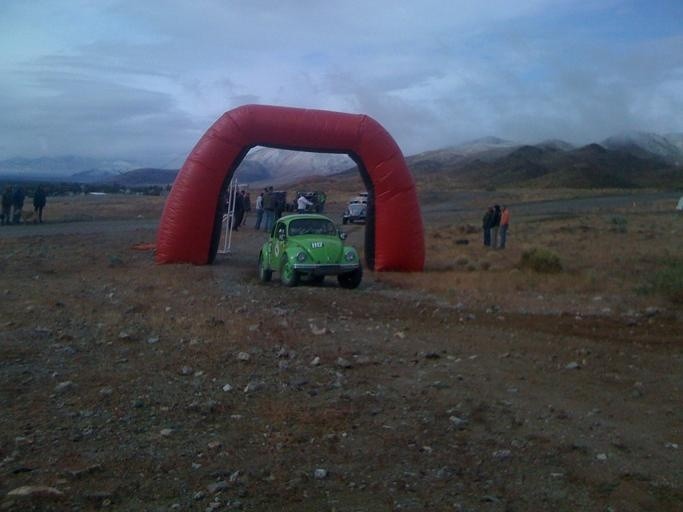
[342,191,368,225]
[293,190,326,212]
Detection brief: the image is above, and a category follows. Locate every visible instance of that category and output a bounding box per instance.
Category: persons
[33,183,46,223]
[222,186,314,234]
[482,204,509,249]
[0,184,25,223]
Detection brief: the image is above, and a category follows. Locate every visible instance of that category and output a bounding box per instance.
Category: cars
[257,213,361,289]
